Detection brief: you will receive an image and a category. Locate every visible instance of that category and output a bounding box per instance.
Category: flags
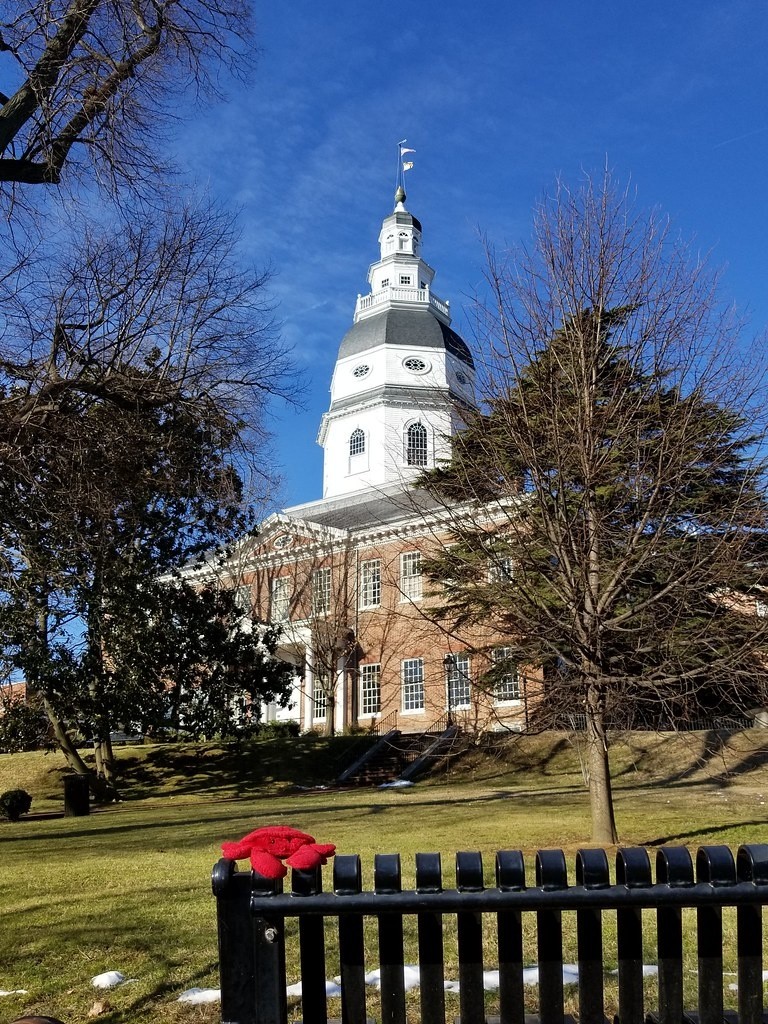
[400,147,416,157]
[402,161,414,171]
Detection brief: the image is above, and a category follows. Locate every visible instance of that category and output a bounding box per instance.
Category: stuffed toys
[219,826,337,878]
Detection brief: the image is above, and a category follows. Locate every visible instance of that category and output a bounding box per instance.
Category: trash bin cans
[61,773,91,817]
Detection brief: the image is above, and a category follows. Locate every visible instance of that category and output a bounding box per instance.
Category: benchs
[86,731,145,746]
